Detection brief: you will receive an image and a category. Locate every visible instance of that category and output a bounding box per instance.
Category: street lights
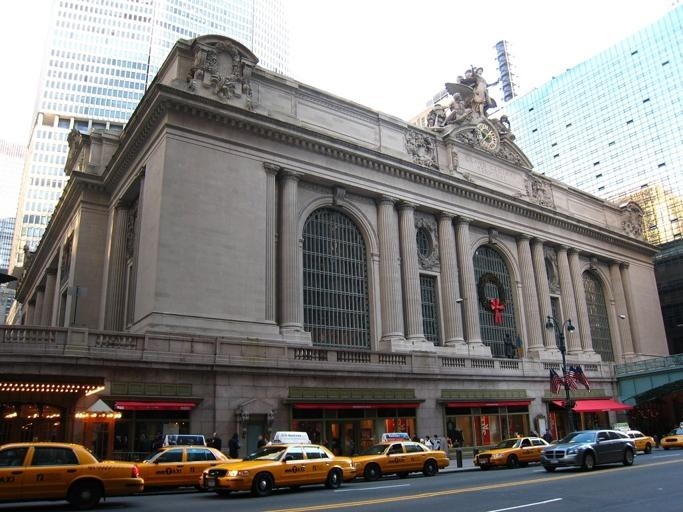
[545,315,575,432]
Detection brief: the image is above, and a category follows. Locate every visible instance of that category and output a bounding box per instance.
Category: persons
[412,433,441,450]
[228,432,240,458]
[207,432,222,451]
[257,434,266,448]
[426,65,533,170]
[503,333,516,359]
[542,428,553,443]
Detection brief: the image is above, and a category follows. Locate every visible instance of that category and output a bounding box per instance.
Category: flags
[549,364,591,394]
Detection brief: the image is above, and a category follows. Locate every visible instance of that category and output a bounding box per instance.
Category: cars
[472,422,683,472]
